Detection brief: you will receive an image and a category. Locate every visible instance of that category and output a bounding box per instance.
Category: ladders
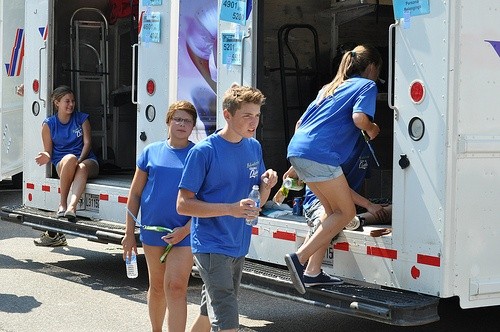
[278,23,320,155]
[70,8,108,159]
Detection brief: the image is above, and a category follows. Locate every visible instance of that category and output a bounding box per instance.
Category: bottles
[246,185,260,227]
[125,249,138,279]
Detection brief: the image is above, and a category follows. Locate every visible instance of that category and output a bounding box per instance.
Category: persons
[35,87,99,220]
[282,43,382,293]
[281,138,387,268]
[123,101,197,332]
[176,85,278,332]
[34,229,68,247]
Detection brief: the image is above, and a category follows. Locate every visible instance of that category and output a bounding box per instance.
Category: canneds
[284,178,304,190]
[293,197,303,216]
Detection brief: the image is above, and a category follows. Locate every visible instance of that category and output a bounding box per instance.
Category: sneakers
[33,231,67,246]
[304,268,344,287]
[285,253,306,295]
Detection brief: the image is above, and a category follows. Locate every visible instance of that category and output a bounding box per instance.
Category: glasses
[172,117,194,127]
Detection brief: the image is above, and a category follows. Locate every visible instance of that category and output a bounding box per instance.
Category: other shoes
[344,215,365,230]
[57,206,64,217]
[65,211,76,222]
